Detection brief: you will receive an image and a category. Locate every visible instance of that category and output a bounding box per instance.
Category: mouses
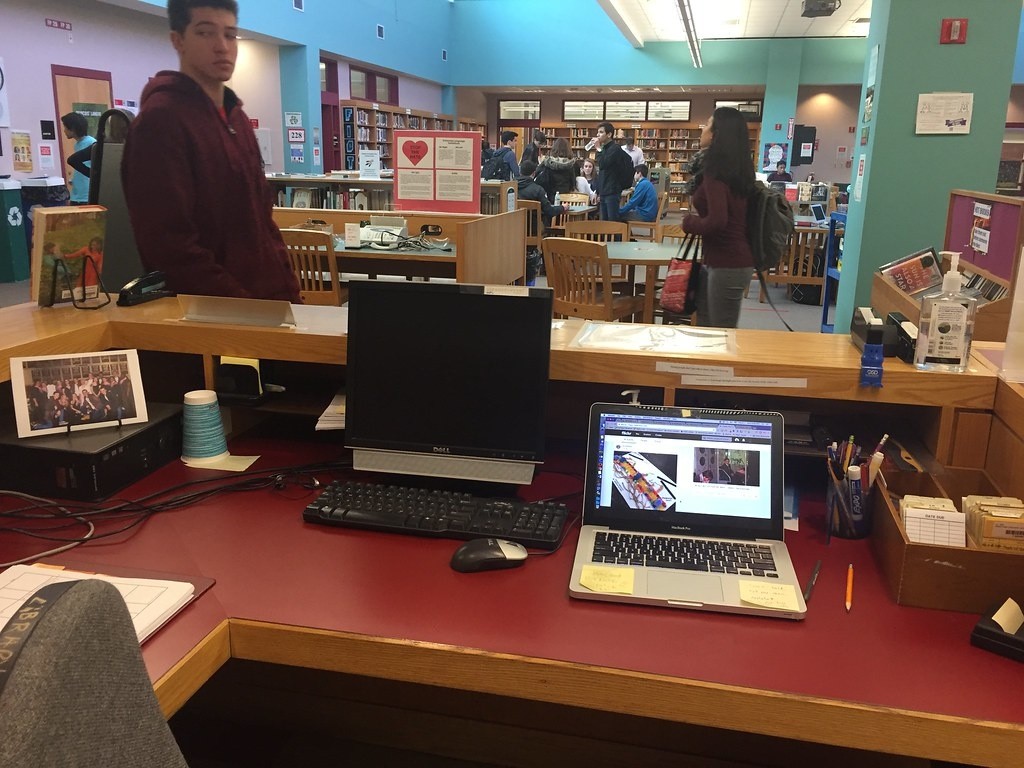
[450,537,529,574]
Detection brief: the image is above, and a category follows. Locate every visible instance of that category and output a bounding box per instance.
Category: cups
[827,479,874,540]
[180,389,229,463]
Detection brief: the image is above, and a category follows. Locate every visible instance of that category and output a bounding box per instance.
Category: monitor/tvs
[812,204,826,225]
[345,283,554,502]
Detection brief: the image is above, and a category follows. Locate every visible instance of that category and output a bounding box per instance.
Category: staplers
[116,270,167,306]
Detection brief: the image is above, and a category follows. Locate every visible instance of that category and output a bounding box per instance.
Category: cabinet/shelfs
[340,99,489,172]
[538,120,760,212]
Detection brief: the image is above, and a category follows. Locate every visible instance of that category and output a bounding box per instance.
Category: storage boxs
[872,466,1024,614]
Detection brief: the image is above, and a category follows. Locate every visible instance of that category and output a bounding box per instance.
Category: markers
[869,451,884,490]
[860,463,869,495]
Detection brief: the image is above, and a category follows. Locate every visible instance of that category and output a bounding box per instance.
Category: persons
[767,161,792,182]
[43,241,79,282]
[480,123,658,277]
[681,107,754,328]
[61,111,132,205]
[14,146,32,162]
[120,0,305,303]
[694,459,747,484]
[64,237,103,290]
[27,372,131,428]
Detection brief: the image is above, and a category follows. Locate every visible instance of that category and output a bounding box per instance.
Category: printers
[359,215,408,246]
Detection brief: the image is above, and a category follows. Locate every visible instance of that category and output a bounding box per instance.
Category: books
[32,205,108,304]
[355,108,485,170]
[541,127,700,205]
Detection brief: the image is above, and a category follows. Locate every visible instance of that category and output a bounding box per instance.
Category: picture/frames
[11,349,150,441]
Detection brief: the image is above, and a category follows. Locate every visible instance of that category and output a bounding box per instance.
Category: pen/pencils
[827,435,861,536]
[846,563,853,611]
[804,560,822,600]
[868,434,889,463]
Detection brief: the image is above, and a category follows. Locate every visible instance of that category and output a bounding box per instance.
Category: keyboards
[302,478,569,551]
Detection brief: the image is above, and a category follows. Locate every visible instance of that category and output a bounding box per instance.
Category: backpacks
[608,143,635,190]
[787,253,824,306]
[482,148,510,180]
[748,179,794,269]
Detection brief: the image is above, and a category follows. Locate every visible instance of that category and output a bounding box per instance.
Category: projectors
[800,0,836,18]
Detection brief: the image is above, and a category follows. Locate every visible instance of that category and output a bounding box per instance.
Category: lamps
[676,0,703,69]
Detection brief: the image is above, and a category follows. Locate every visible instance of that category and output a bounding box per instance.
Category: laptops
[569,402,808,620]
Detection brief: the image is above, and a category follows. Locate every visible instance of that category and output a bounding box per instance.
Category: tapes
[859,307,883,325]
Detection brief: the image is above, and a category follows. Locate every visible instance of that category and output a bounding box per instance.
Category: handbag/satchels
[534,159,551,191]
[657,234,702,315]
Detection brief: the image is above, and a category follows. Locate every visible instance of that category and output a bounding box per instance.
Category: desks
[774,215,833,288]
[584,241,704,325]
[273,209,529,283]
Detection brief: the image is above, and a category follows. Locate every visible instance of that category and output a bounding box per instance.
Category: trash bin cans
[0,179,31,283]
[21,176,66,267]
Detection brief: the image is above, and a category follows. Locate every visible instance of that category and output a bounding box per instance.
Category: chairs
[541,237,647,324]
[634,223,702,326]
[280,227,350,308]
[563,219,627,322]
[626,192,668,241]
[518,200,543,256]
[546,193,591,238]
